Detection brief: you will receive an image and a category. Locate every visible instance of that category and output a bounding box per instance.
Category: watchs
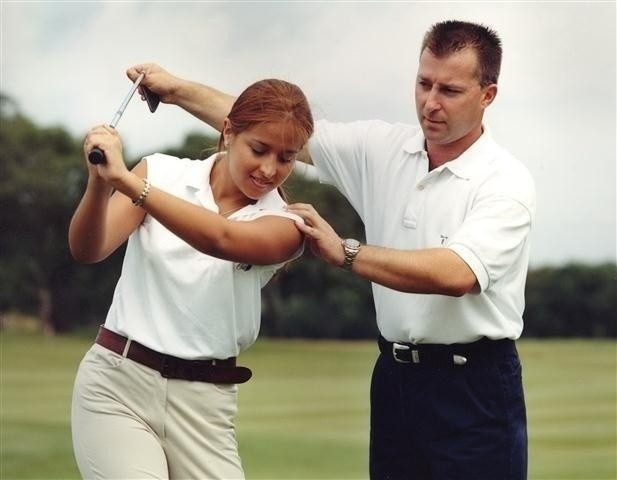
[337,236,361,274]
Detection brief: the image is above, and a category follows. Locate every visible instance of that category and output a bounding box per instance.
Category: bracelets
[129,176,152,207]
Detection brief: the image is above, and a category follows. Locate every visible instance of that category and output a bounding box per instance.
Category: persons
[123,19,537,479]
[67,77,315,480]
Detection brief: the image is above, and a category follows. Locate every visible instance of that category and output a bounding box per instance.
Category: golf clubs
[88,71,158,165]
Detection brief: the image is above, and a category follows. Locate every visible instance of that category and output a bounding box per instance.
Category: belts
[94,325,252,383]
[378,334,514,366]
